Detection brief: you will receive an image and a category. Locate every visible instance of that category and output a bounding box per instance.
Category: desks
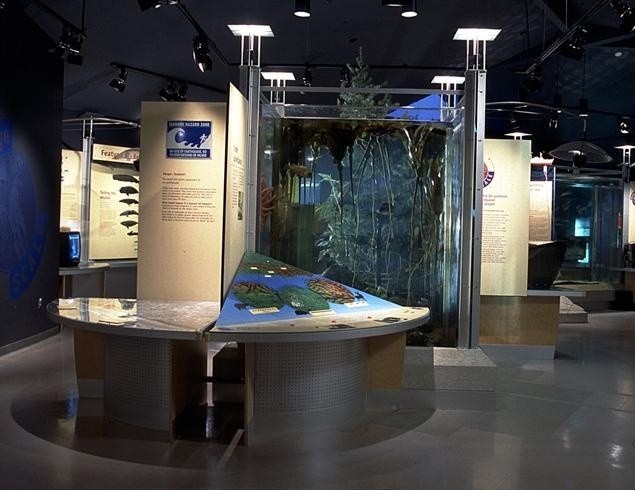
[57,262,111,335]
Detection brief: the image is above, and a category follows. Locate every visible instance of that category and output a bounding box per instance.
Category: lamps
[262,72,298,103]
[388,2,424,20]
[453,26,502,72]
[51,30,87,69]
[431,74,464,108]
[293,2,314,19]
[104,67,130,98]
[159,80,189,103]
[505,4,635,169]
[190,33,216,77]
[224,20,275,67]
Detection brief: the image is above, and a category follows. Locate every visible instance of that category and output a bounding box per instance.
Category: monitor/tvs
[60,231,81,268]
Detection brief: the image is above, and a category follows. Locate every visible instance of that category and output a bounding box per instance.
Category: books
[97,316,137,324]
[59,297,78,310]
